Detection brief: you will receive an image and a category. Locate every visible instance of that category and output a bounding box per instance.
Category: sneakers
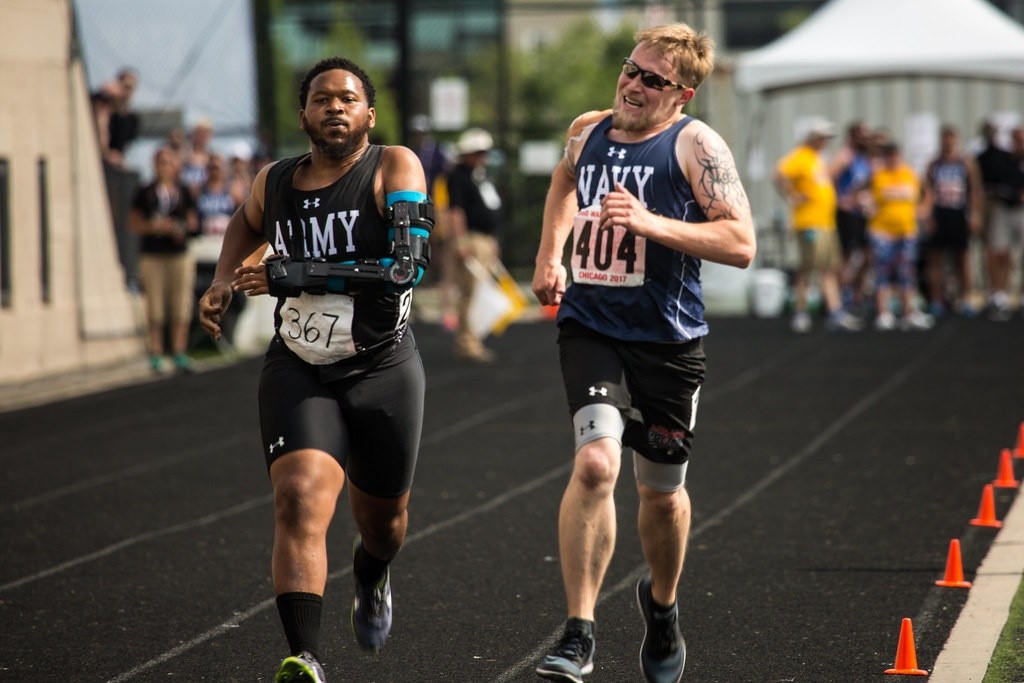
[637,574,686,682]
[273,652,325,683]
[535,617,596,683]
[350,533,392,653]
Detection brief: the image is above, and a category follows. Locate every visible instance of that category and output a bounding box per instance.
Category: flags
[484,276,529,338]
[466,275,510,338]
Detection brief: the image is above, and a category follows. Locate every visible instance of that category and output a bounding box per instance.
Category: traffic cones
[992,450,1022,488]
[970,485,1003,529]
[935,539,972,589]
[885,618,930,677]
[1014,422,1024,457]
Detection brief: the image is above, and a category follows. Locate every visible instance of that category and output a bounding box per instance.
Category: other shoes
[792,287,1022,334]
[173,356,198,375]
[148,360,170,377]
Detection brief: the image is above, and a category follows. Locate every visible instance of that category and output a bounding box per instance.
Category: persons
[770,117,1024,332]
[200,57,435,683]
[534,19,757,683]
[93,66,506,365]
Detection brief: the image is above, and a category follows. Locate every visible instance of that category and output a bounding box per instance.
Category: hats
[802,118,836,138]
[458,128,493,153]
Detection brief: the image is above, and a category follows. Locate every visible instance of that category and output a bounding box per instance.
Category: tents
[730,0,1024,253]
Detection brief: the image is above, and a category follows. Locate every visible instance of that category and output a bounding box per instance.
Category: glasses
[623,57,686,91]
